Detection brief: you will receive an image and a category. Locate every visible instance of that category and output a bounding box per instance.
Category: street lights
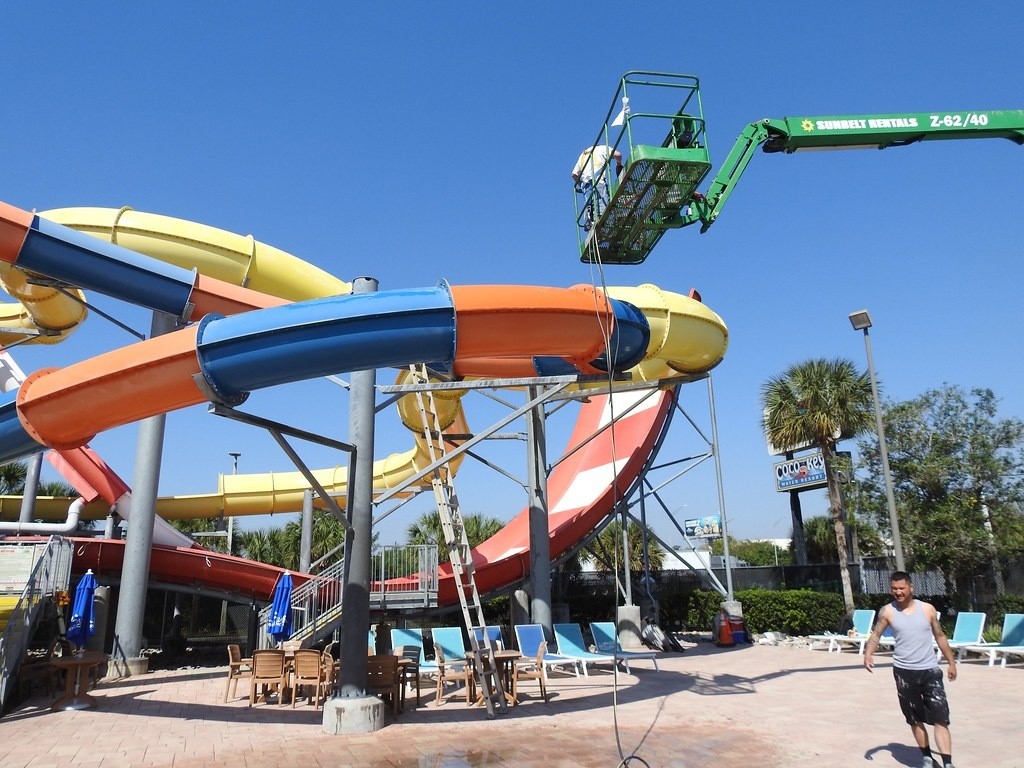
[848,308,907,573]
[667,504,689,550]
[771,515,787,567]
[219,451,241,636]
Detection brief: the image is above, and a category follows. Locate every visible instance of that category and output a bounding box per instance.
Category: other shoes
[922,756,941,768]
[945,763,956,768]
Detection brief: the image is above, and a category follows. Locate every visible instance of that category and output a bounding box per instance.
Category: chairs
[809,608,1023,669]
[224,621,660,706]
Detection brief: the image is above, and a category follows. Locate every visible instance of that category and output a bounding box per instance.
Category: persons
[571,144,623,235]
[864,571,957,768]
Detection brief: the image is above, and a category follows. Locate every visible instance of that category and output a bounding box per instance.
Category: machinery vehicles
[572,68,1024,266]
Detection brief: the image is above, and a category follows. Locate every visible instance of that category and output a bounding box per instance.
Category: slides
[1,204,733,525]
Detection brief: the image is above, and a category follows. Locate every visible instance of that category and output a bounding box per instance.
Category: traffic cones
[716,608,736,648]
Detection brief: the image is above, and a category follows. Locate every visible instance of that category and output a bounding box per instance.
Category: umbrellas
[65,568,98,696]
[266,569,294,688]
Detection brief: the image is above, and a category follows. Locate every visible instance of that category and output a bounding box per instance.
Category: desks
[50,651,112,711]
[396,657,414,714]
[255,656,295,704]
[464,649,522,707]
